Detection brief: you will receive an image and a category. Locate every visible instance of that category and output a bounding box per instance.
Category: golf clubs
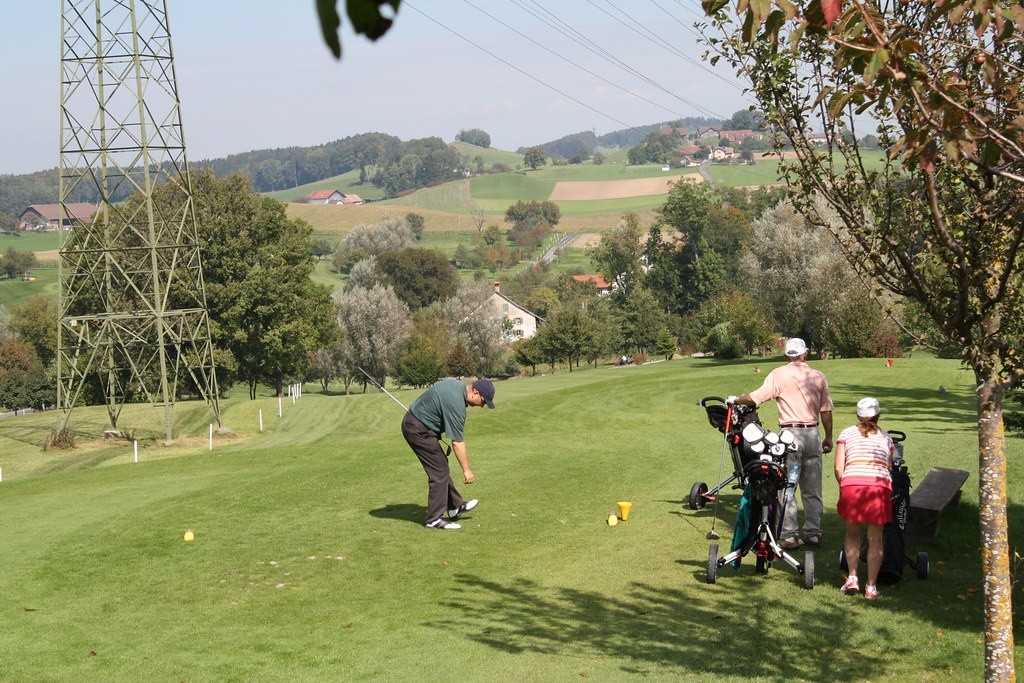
[706,404,733,540]
[357,366,452,456]
[742,421,799,476]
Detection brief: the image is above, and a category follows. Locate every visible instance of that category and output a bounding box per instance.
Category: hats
[856,397,880,417]
[472,379,495,408]
[784,337,809,357]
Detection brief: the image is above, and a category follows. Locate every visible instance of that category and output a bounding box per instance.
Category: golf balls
[608,515,618,525]
[184,532,194,541]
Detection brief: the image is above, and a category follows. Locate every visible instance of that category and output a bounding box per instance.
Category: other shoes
[797,534,823,546]
[776,536,799,548]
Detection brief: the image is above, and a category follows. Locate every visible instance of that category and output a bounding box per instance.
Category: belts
[781,423,818,427]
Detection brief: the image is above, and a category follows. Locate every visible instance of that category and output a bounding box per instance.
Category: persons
[400,377,495,529]
[833,396,895,600]
[724,338,834,551]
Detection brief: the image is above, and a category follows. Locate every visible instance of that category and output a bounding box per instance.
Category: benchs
[909,466,969,538]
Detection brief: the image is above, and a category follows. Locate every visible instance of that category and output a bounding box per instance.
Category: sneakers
[840,576,859,592]
[447,499,478,520]
[865,587,880,599]
[426,518,461,529]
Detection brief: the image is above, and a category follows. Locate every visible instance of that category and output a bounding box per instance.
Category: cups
[616,502,632,521]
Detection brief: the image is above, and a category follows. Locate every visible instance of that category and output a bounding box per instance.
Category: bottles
[786,466,799,497]
[607,511,618,526]
[183,529,197,541]
